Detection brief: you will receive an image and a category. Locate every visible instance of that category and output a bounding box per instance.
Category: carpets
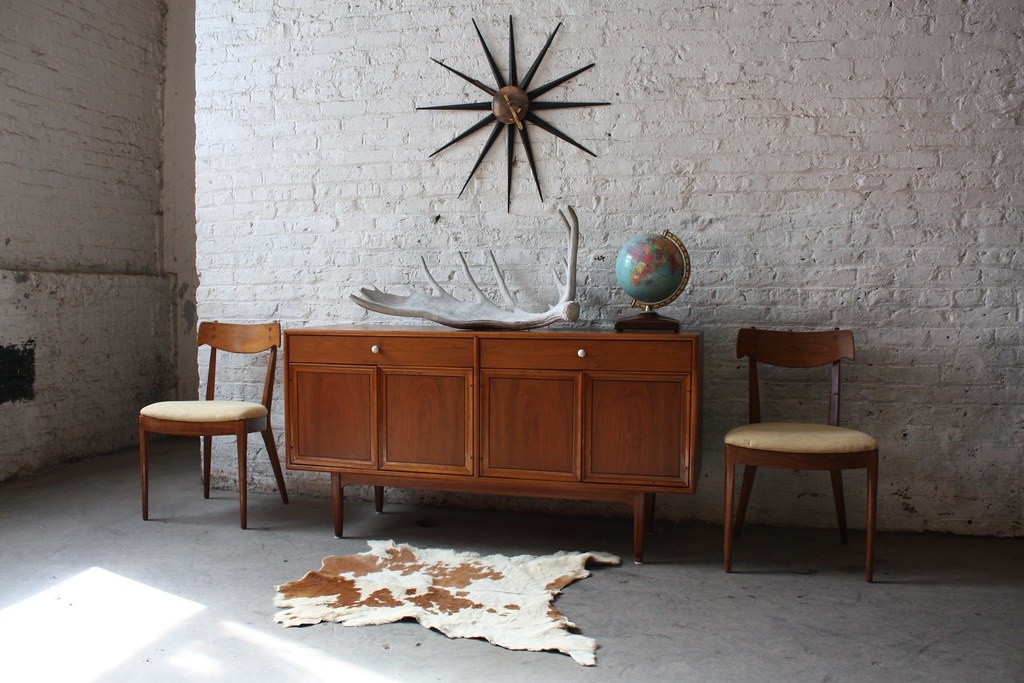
[277,539,622,662]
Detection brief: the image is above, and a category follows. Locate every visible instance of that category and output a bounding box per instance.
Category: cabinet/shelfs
[284,323,705,565]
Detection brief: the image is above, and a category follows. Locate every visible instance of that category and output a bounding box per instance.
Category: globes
[613,230,692,333]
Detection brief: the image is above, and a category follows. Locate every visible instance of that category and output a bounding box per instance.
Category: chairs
[725,325,878,582]
[140,320,290,530]
